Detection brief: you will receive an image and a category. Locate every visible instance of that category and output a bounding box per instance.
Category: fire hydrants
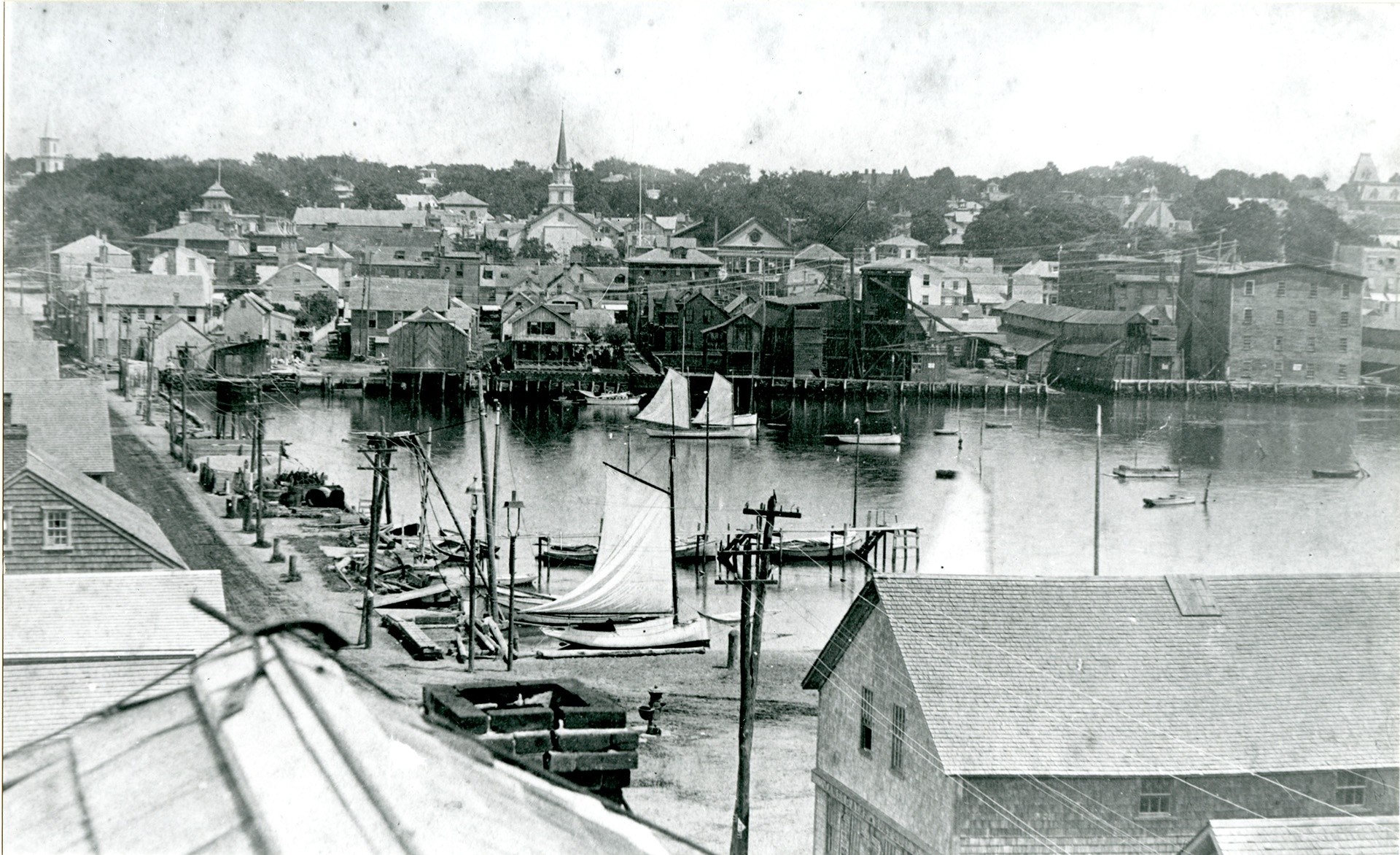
[648,684,666,720]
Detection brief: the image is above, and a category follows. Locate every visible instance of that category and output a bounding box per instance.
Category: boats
[545,542,599,562]
[822,434,901,445]
[986,420,1012,428]
[772,532,861,559]
[1312,467,1360,478]
[933,429,959,436]
[357,498,419,536]
[431,529,500,560]
[456,575,534,587]
[1112,464,1176,480]
[1143,493,1193,507]
[578,393,645,405]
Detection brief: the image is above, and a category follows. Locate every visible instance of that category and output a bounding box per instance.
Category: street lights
[463,476,486,671]
[502,491,524,670]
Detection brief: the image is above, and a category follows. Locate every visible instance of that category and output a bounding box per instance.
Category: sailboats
[634,314,757,438]
[492,379,791,648]
